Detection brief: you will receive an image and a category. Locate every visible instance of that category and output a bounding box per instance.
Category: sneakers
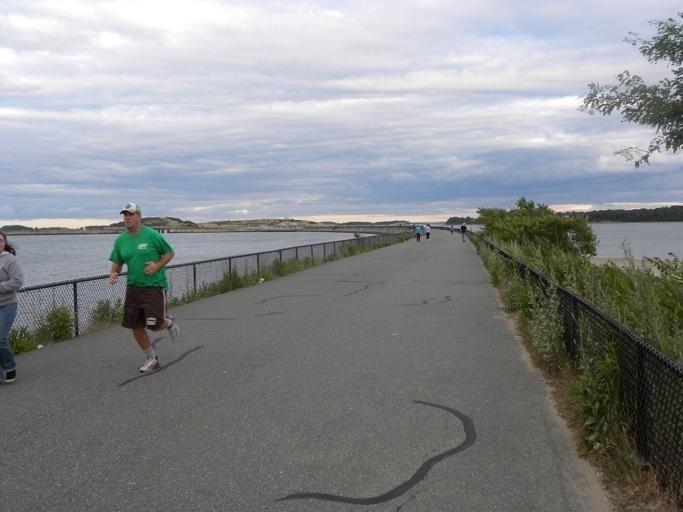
[4,368,16,383]
[166,315,179,342]
[139,355,160,373]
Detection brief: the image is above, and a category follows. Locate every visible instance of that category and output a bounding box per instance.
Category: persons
[425,223,431,240]
[0,234,23,384]
[106,202,181,374]
[415,225,421,244]
[459,223,467,242]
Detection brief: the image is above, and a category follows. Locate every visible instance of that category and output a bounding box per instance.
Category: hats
[120,203,141,216]
